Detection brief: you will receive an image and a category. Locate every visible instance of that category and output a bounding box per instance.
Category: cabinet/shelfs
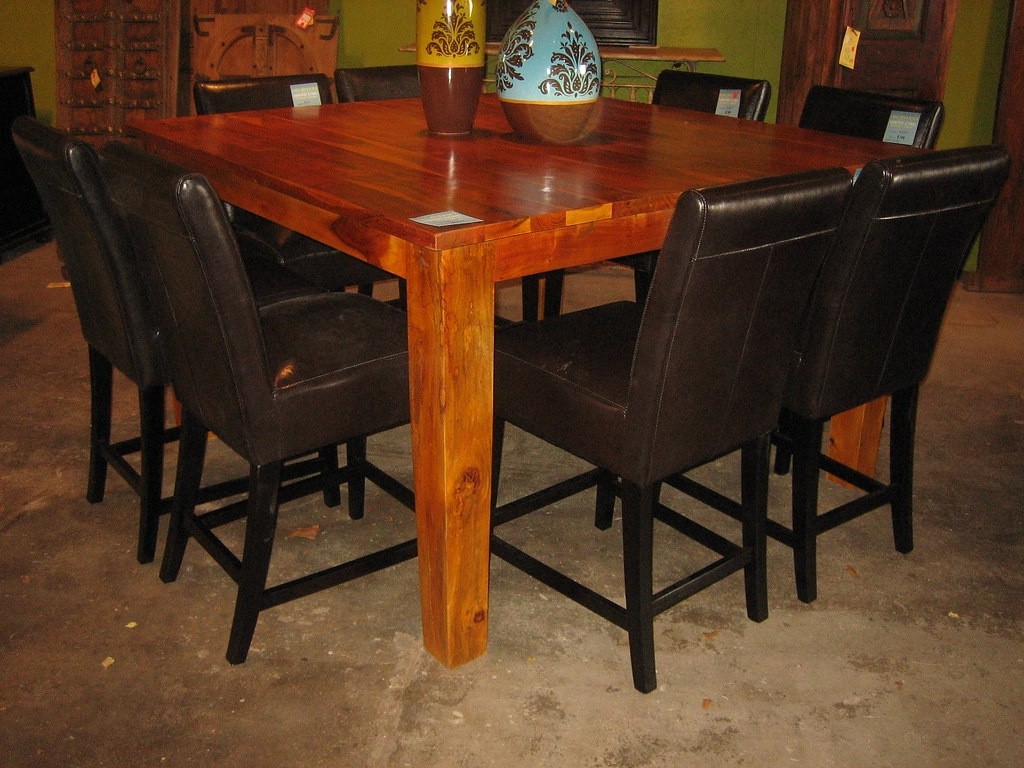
[0,65,53,257]
[52,1,340,152]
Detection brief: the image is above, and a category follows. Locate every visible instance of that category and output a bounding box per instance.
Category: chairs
[8,73,418,663]
[488,66,1015,695]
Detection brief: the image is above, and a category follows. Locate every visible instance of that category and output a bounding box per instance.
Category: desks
[124,99,931,670]
[398,43,726,104]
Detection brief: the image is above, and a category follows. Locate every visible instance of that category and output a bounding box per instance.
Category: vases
[416,0,486,137]
[495,0,601,143]
